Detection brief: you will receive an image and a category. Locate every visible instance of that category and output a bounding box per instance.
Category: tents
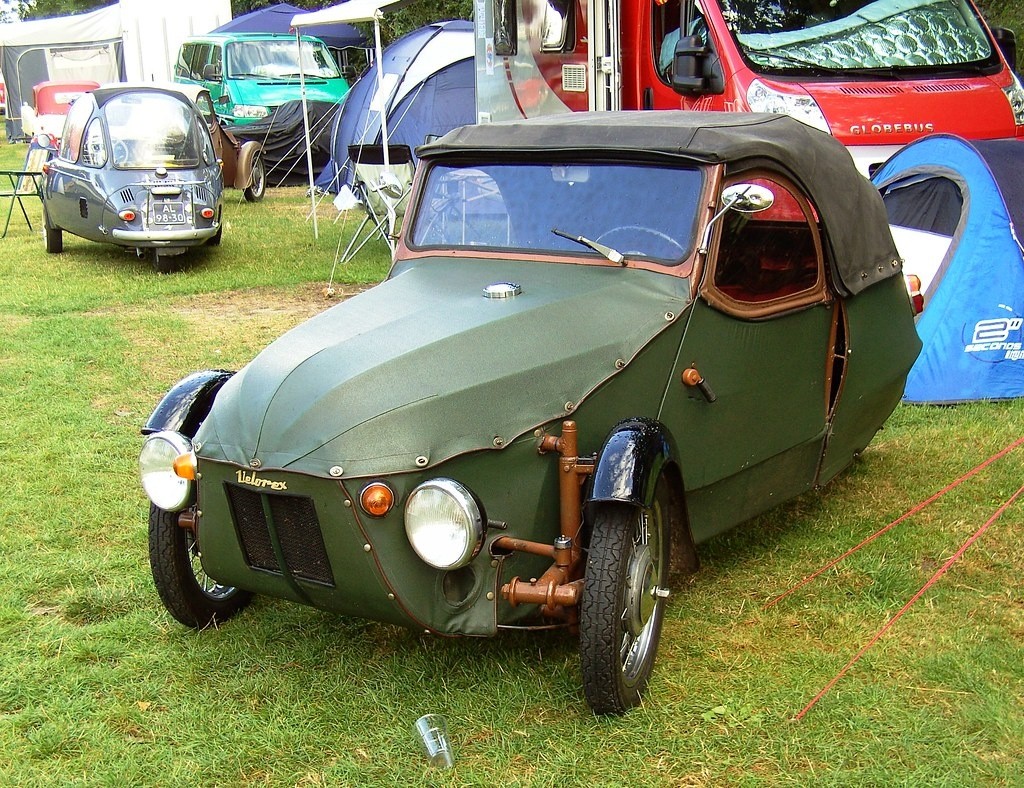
[0,1,477,297]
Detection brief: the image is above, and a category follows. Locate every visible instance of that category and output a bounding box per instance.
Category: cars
[38,81,268,274]
[139,112,924,717]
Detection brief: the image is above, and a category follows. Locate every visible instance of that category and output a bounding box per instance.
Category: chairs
[341,145,418,265]
[0,143,51,240]
[713,226,820,303]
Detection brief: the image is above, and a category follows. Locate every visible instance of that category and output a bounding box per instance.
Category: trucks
[492,0,1024,307]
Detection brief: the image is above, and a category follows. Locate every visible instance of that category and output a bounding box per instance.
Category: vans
[173,32,351,126]
